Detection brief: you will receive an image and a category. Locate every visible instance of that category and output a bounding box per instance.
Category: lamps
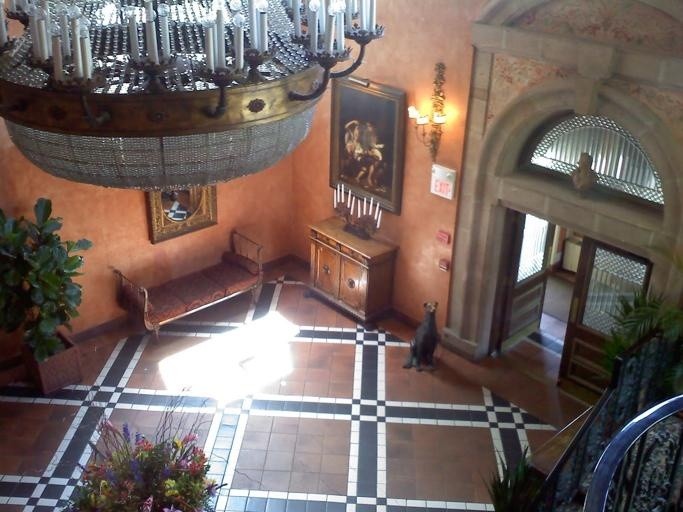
[1,0,386,193]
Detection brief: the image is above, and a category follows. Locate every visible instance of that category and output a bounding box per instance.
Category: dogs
[402,301,442,372]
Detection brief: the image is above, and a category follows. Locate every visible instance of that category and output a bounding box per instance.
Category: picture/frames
[328,76,406,216]
[148,184,218,245]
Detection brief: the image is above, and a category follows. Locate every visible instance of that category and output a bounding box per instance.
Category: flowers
[55,383,228,512]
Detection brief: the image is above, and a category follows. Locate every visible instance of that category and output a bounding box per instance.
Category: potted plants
[1,198,94,395]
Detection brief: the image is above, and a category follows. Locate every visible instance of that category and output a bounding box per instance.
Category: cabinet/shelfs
[303,216,400,329]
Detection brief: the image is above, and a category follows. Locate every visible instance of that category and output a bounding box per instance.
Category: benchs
[113,229,265,347]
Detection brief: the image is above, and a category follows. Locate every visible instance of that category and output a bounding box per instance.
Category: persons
[344,119,382,188]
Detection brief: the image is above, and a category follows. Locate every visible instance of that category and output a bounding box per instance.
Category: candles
[332,182,382,229]
